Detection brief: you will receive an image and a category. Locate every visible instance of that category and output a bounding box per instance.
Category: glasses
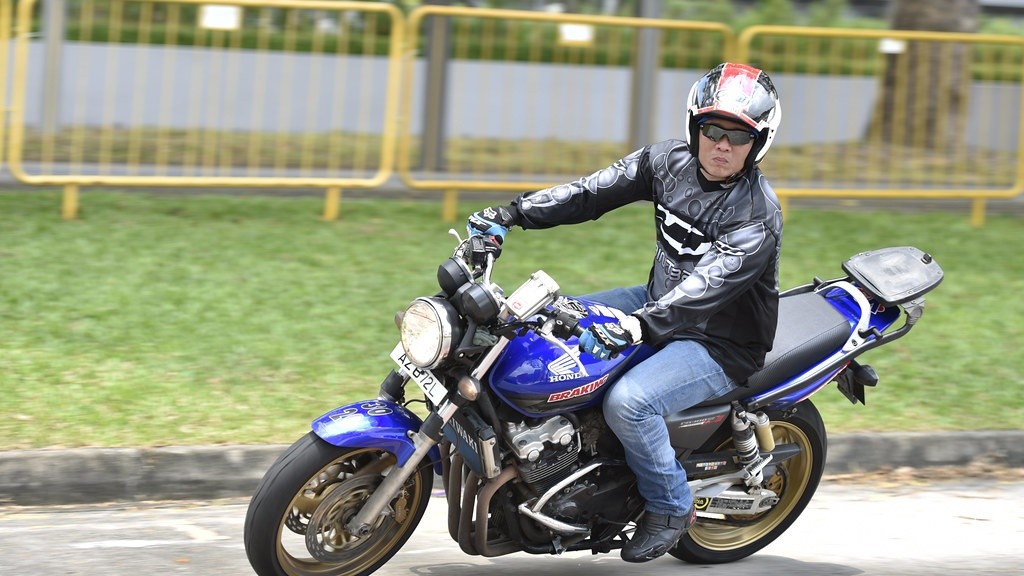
[700,121,756,146]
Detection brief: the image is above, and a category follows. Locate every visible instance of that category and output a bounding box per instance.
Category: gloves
[577,322,635,362]
[466,205,514,246]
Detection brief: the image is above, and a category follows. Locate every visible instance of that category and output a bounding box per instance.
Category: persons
[467,62,786,564]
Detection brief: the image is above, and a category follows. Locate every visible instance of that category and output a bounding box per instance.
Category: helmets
[685,62,782,172]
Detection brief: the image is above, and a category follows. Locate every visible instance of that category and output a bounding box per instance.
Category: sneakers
[620,500,700,563]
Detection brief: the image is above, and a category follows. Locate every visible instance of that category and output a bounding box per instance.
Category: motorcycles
[242,222,945,576]
